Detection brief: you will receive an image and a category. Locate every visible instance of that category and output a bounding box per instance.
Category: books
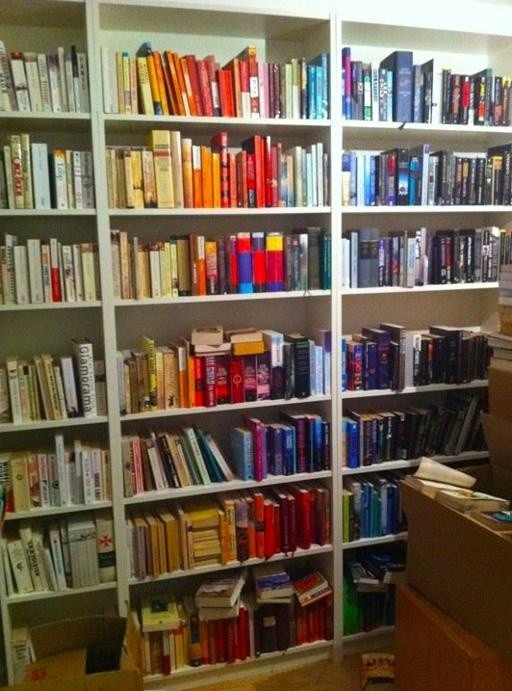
[488,265,512,358]
[343,144,511,206]
[359,653,395,688]
[1,508,116,596]
[342,47,512,126]
[101,41,328,118]
[126,480,331,580]
[106,130,329,209]
[11,620,31,681]
[342,392,488,469]
[0,40,89,112]
[0,430,112,511]
[0,233,101,304]
[110,226,331,298]
[0,337,107,423]
[0,132,94,210]
[104,564,334,677]
[117,324,332,414]
[341,457,511,637]
[122,410,330,497]
[342,228,511,288]
[341,323,494,392]
